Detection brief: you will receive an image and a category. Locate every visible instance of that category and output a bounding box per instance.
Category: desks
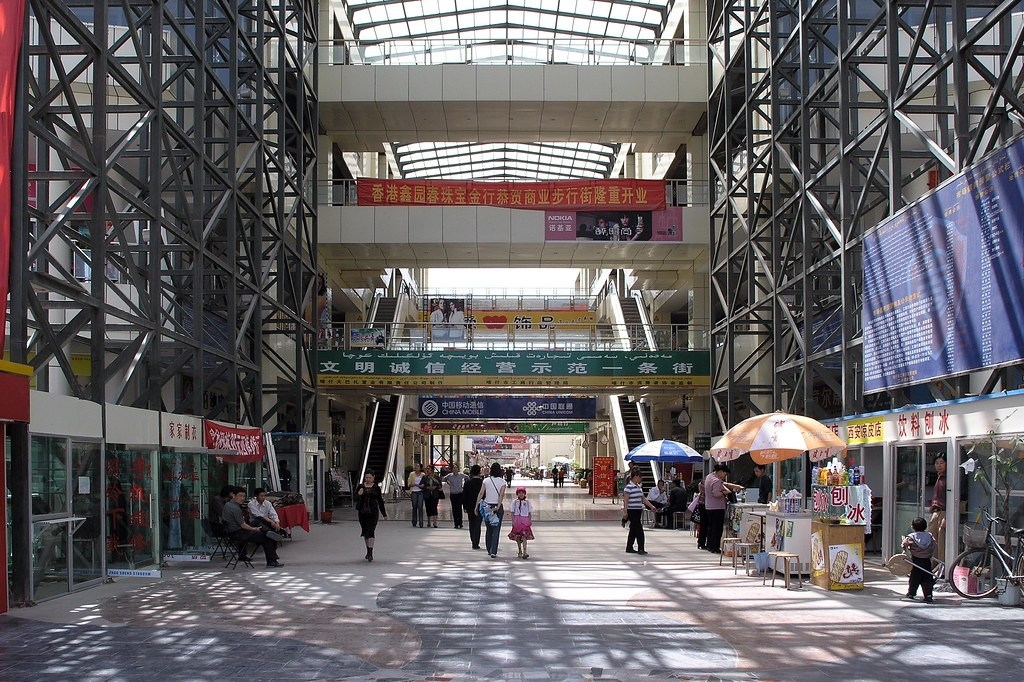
[275,502,310,544]
[386,336,431,351]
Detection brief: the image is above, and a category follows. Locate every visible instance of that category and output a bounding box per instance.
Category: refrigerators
[719,501,770,556]
[766,510,811,576]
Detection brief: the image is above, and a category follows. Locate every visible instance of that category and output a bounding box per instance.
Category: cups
[827,457,842,473]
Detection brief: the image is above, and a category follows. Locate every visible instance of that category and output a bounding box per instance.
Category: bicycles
[948,504,1024,603]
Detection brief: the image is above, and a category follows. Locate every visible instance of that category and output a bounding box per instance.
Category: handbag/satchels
[356,484,363,510]
[438,489,445,499]
[690,504,700,524]
[728,491,737,503]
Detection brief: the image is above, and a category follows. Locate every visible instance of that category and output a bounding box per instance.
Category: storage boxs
[953,565,978,594]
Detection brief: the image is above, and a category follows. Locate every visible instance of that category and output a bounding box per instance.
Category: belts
[933,509,943,511]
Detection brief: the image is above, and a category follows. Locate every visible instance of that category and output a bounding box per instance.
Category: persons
[621,462,640,528]
[430,300,464,328]
[928,451,947,578]
[462,464,486,549]
[559,468,564,488]
[662,478,687,529]
[552,465,558,488]
[753,463,773,504]
[247,487,288,559]
[407,463,425,528]
[208,485,284,568]
[463,463,544,488]
[644,480,668,528]
[278,460,292,491]
[443,464,469,529]
[623,469,657,555]
[593,213,644,241]
[353,468,388,562]
[474,462,508,558]
[507,486,535,559]
[698,463,745,553]
[419,465,443,528]
[900,516,937,604]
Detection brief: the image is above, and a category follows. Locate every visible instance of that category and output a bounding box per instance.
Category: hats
[517,486,526,491]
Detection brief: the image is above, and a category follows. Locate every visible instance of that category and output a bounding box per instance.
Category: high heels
[697,543,706,550]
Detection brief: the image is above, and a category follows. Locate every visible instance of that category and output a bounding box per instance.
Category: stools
[641,507,654,526]
[720,536,745,567]
[672,511,687,532]
[734,541,760,576]
[771,551,803,591]
[391,342,403,351]
[414,342,423,351]
[762,550,792,587]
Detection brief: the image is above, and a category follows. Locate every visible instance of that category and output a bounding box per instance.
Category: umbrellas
[709,409,847,503]
[551,457,572,464]
[624,438,704,480]
[538,466,546,469]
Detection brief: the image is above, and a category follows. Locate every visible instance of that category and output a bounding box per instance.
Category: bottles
[741,492,745,503]
[812,465,864,487]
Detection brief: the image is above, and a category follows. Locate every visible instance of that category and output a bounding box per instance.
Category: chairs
[220,518,257,570]
[205,516,237,561]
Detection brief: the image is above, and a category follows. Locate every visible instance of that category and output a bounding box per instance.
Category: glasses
[937,452,945,458]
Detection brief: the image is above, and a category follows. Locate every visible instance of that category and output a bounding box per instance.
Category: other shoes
[472,545,480,549]
[626,549,638,553]
[460,525,463,528]
[266,530,282,541]
[413,523,416,527]
[638,550,647,554]
[523,554,529,559]
[664,526,673,529]
[433,523,437,528]
[365,555,368,559]
[518,553,522,558]
[454,526,458,528]
[923,597,933,603]
[906,593,913,599]
[266,562,284,568]
[427,524,430,527]
[490,554,497,557]
[708,547,724,553]
[655,524,662,528]
[420,525,423,527]
[621,519,625,527]
[368,556,373,561]
